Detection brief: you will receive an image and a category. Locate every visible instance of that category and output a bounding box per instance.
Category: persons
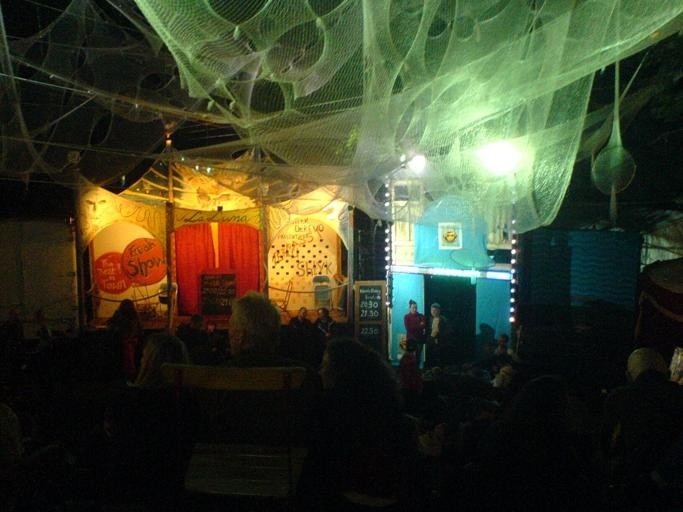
[113,299,136,324]
[229,292,286,370]
[402,298,427,364]
[290,307,312,328]
[314,308,339,336]
[427,303,454,370]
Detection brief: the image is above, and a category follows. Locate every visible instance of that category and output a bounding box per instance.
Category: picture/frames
[437,221,462,250]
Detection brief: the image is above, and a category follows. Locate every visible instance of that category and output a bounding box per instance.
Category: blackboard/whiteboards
[201,273,238,316]
[354,280,389,361]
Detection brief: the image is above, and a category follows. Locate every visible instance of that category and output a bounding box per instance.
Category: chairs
[169,356,311,509]
